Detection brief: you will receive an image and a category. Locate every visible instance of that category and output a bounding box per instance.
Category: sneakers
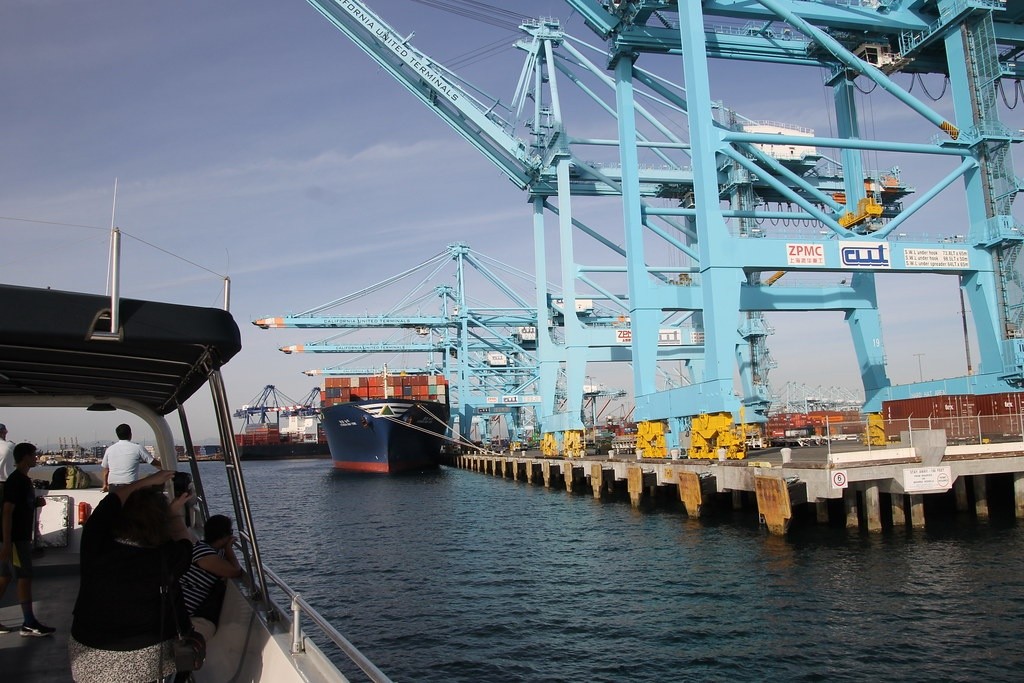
[0,625,11,633]
[19,622,56,637]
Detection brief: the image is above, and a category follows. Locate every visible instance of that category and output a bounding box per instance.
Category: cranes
[232,1,1023,463]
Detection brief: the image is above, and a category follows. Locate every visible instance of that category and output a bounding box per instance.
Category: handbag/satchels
[172,631,207,671]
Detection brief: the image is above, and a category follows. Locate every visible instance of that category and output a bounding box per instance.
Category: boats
[320,363,450,473]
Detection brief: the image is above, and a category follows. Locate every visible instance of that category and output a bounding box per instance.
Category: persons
[69,470,242,683]
[0,423,56,637]
[101,424,161,493]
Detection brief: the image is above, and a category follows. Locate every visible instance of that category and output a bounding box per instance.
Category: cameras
[172,472,193,497]
